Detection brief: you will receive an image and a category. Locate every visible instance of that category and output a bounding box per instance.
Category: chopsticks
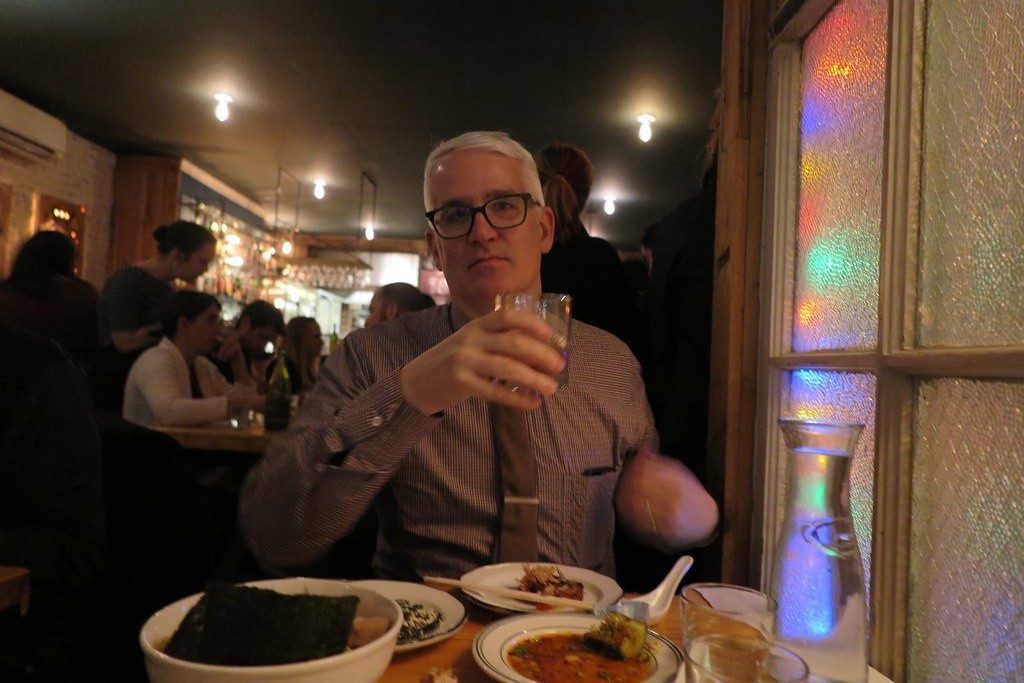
[424,575,596,612]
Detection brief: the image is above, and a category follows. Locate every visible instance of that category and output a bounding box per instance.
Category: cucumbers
[584,611,647,659]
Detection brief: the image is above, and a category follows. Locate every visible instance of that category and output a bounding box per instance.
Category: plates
[472,613,685,683]
[348,578,468,650]
[460,562,623,616]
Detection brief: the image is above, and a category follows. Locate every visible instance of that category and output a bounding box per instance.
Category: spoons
[627,555,694,622]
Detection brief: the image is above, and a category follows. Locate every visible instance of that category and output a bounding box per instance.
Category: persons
[242,133,722,581]
[366,283,435,327]
[1,222,323,683]
[535,143,626,342]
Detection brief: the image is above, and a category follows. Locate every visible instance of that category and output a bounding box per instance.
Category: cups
[494,292,573,391]
[227,392,254,429]
[682,582,777,682]
[683,638,810,682]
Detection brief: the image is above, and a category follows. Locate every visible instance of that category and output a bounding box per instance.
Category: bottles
[328,324,338,351]
[263,348,291,431]
[767,416,870,682]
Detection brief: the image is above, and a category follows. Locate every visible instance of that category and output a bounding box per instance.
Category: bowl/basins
[139,576,402,683]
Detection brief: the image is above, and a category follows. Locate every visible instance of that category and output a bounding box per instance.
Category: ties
[187,363,205,398]
[485,381,543,564]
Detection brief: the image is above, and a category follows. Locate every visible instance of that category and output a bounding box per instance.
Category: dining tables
[380,587,899,683]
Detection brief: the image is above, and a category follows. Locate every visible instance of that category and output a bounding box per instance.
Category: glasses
[423,191,543,241]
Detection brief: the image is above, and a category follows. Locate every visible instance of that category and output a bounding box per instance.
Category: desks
[154,424,291,450]
